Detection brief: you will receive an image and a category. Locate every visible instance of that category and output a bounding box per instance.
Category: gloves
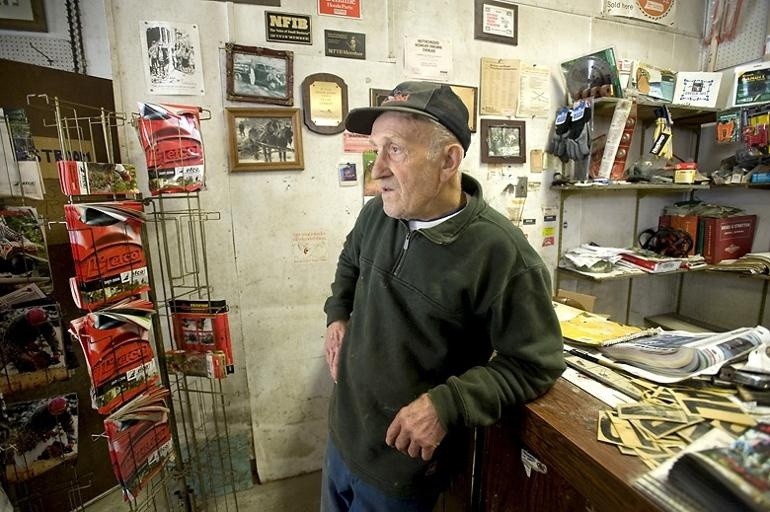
[653,107,674,160]
[549,107,590,163]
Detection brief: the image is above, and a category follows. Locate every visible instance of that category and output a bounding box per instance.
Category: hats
[344,80,471,158]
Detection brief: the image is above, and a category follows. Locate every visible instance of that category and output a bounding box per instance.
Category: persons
[320,82,567,512]
[4,309,62,374]
[239,121,245,138]
[17,399,77,461]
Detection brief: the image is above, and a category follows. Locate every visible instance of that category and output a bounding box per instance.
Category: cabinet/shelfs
[552,91,770,329]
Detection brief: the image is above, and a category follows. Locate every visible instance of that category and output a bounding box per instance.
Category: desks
[444,353,770,511]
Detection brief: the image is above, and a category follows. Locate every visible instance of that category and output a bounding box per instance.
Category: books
[56,102,235,503]
[619,202,756,274]
[556,313,770,380]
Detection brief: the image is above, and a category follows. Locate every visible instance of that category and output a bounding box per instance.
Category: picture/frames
[223,41,295,106]
[0,0,49,32]
[473,0,519,46]
[672,71,724,109]
[223,104,304,173]
[480,118,526,164]
